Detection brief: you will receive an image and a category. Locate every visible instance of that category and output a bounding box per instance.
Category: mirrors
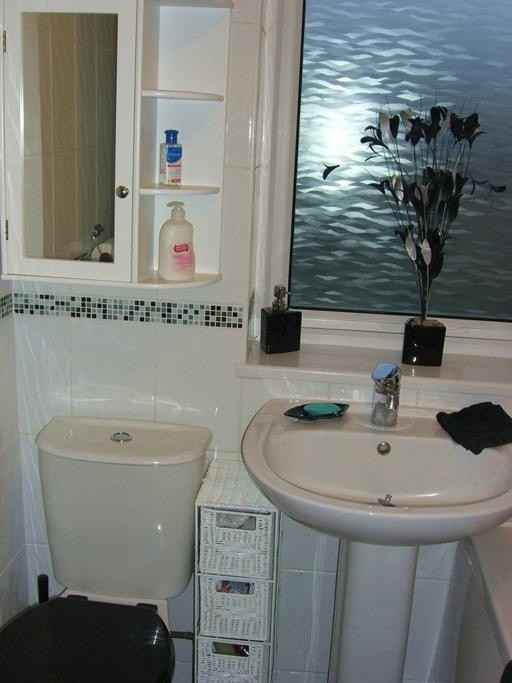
[3,4,136,285]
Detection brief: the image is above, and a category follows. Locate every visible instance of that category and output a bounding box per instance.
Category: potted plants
[319,89,506,367]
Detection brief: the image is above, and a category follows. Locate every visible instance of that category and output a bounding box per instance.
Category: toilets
[0,417,213,683]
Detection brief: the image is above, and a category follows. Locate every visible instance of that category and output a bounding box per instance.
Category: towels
[436,401,511,455]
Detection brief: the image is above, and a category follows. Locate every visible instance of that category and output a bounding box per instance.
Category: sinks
[240,396,512,543]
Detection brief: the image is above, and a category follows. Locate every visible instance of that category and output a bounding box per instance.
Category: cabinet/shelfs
[192,456,281,683]
[137,3,231,290]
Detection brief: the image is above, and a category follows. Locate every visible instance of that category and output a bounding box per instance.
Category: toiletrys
[158,128,182,186]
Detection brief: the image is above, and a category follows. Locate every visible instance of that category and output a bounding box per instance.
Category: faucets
[372,361,400,426]
